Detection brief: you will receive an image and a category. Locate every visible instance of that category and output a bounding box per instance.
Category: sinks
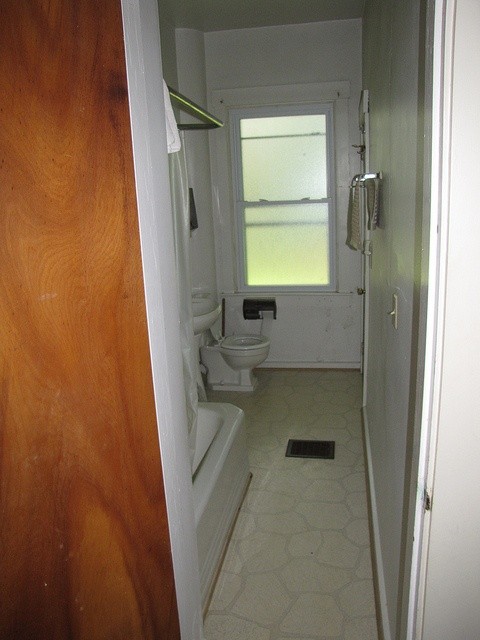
[191,299,223,336]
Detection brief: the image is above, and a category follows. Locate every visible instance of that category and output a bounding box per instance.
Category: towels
[345,173,380,254]
[162,77,182,156]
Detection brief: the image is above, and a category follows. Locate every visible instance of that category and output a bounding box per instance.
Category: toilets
[193,292,271,392]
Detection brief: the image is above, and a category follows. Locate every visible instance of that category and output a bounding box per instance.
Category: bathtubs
[191,401,253,621]
